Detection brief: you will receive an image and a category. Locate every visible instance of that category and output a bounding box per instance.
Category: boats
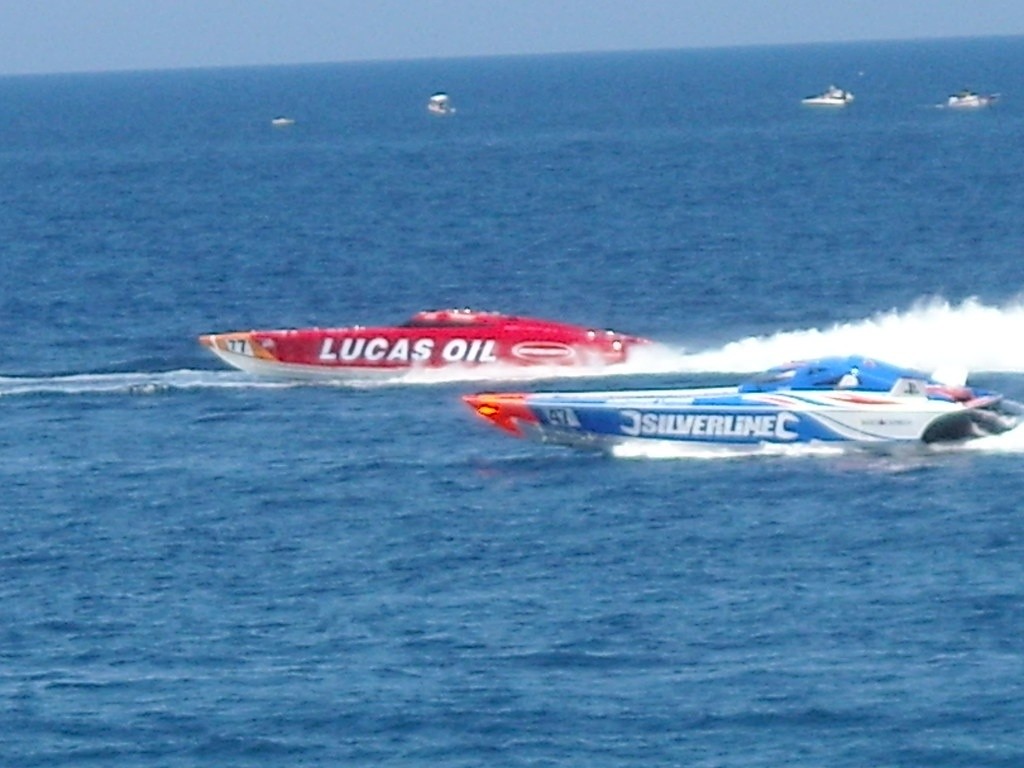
[469,352,1023,454]
[198,309,650,387]
